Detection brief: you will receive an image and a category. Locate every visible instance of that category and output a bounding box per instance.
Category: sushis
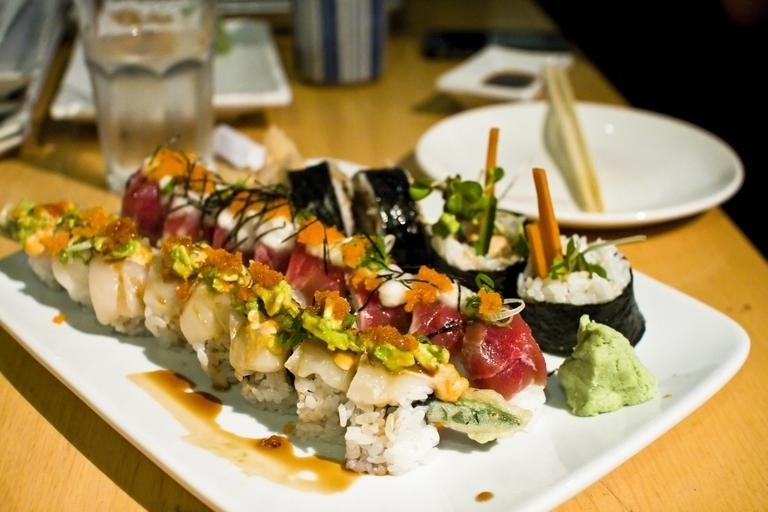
[0,127,647,475]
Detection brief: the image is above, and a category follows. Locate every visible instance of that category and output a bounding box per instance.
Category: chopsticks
[541,60,604,216]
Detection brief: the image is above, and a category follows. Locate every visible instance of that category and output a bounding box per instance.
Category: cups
[292,0,379,85]
[71,0,216,196]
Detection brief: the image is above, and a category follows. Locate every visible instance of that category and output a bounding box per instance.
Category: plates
[49,16,292,126]
[0,161,750,512]
[413,94,747,231]
[433,44,575,110]
